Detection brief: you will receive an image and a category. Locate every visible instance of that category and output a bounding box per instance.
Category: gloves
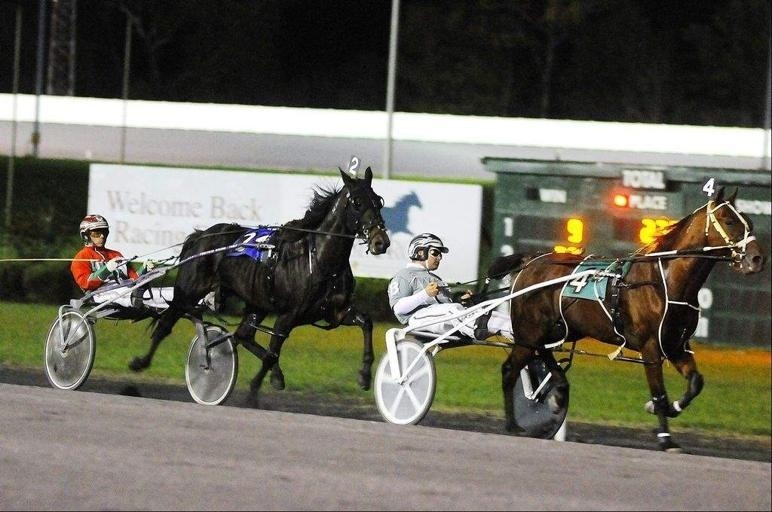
[106,255,125,272]
[137,259,154,277]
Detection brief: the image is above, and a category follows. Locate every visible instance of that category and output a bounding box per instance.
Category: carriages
[40,163,392,407]
[372,184,766,457]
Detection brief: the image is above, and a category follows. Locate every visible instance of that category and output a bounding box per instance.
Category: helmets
[79,214,109,238]
[408,233,449,261]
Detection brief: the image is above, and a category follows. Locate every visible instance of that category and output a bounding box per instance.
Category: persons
[386,231,565,352]
[67,212,223,315]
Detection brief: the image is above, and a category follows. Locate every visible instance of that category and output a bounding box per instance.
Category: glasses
[429,248,443,260]
[89,228,110,239]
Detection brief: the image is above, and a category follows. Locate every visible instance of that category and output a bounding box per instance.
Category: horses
[485,186,768,456]
[124,165,392,393]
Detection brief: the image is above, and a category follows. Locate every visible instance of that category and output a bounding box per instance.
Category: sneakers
[204,284,222,313]
